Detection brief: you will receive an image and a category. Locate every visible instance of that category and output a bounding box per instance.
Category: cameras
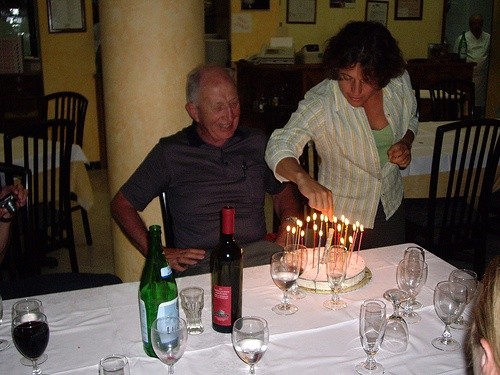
[0,192,19,215]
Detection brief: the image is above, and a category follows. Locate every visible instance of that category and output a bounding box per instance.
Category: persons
[109,61,301,280]
[0,184,28,263]
[454,14,491,106]
[264,20,419,250]
[470,258,500,375]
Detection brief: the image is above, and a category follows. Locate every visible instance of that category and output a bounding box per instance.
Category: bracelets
[0,217,13,223]
[281,217,291,220]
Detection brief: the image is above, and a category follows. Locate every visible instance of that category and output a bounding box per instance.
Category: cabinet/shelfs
[234,62,334,134]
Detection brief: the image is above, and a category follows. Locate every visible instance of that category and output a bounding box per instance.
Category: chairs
[405,58,499,280]
[0,74,93,275]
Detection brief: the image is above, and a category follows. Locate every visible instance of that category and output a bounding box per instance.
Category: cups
[179,287,205,336]
[98,354,131,375]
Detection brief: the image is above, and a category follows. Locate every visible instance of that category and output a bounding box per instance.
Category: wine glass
[431,280,467,354]
[448,268,479,331]
[395,258,429,324]
[397,246,424,308]
[232,316,270,374]
[0,297,10,351]
[11,298,50,375]
[322,245,350,312]
[379,288,416,354]
[354,299,387,375]
[270,252,299,316]
[150,317,188,375]
[281,244,308,300]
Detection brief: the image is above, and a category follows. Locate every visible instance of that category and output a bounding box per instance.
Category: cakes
[279,247,365,290]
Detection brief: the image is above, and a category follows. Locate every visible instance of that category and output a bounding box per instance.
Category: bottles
[458,30,468,63]
[209,205,245,335]
[138,224,180,359]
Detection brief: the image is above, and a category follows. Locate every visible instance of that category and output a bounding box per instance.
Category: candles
[285,212,364,278]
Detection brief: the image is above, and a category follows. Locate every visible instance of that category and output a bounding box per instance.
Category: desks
[0,243,484,375]
[0,133,94,246]
[400,121,500,199]
